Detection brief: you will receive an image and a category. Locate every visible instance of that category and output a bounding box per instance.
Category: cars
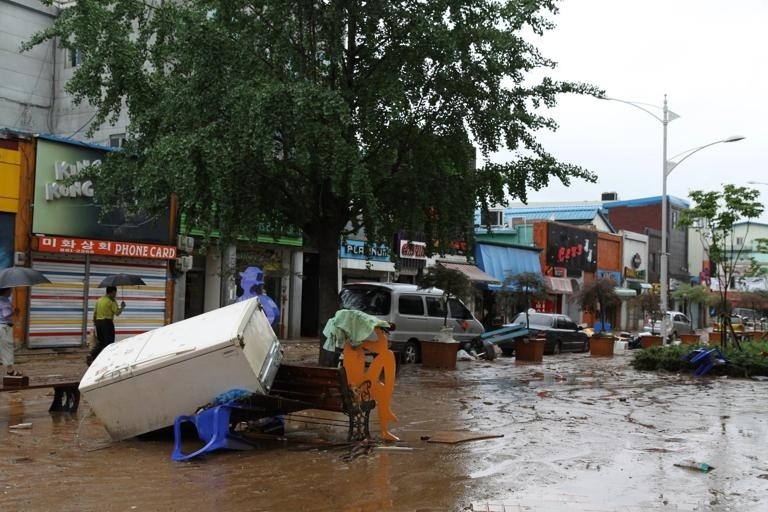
[494,311,590,356]
[643,310,697,341]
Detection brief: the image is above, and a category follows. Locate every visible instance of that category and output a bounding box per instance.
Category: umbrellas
[96,270,146,303]
[0,264,52,308]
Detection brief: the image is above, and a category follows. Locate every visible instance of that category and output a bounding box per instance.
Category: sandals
[7,370,22,376]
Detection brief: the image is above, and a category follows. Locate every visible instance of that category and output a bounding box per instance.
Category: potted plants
[415,258,485,372]
[490,270,560,361]
[564,272,735,359]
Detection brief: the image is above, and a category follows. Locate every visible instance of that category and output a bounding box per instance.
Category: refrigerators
[77,296,284,444]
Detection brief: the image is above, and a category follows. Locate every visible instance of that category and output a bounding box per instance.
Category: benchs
[230,366,378,447]
[1,381,80,413]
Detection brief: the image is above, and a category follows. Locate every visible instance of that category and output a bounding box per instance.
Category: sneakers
[87,356,94,367]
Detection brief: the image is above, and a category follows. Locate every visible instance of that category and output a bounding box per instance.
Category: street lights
[592,90,746,310]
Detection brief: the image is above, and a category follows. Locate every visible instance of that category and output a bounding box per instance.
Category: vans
[337,281,486,365]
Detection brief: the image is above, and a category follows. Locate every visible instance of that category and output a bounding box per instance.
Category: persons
[84,285,125,366]
[235,266,280,328]
[0,287,23,377]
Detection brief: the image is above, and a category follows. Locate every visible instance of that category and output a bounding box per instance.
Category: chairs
[171,399,267,462]
